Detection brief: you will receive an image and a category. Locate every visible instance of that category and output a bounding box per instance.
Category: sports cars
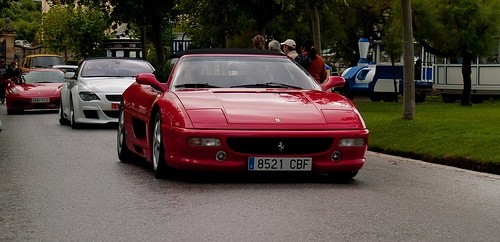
[117,54,371,183]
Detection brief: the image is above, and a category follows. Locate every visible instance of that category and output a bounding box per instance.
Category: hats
[281,39,296,49]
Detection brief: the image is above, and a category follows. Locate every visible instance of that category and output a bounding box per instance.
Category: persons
[295,39,328,84]
[280,39,299,60]
[0,59,17,104]
[253,35,265,50]
[268,40,284,54]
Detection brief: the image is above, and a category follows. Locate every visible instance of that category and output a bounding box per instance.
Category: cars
[59,58,157,130]
[4,68,65,115]
[53,64,79,80]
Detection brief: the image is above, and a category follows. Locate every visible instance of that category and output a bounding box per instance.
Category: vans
[22,54,63,68]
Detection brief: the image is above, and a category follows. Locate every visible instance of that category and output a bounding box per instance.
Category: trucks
[339,38,497,104]
